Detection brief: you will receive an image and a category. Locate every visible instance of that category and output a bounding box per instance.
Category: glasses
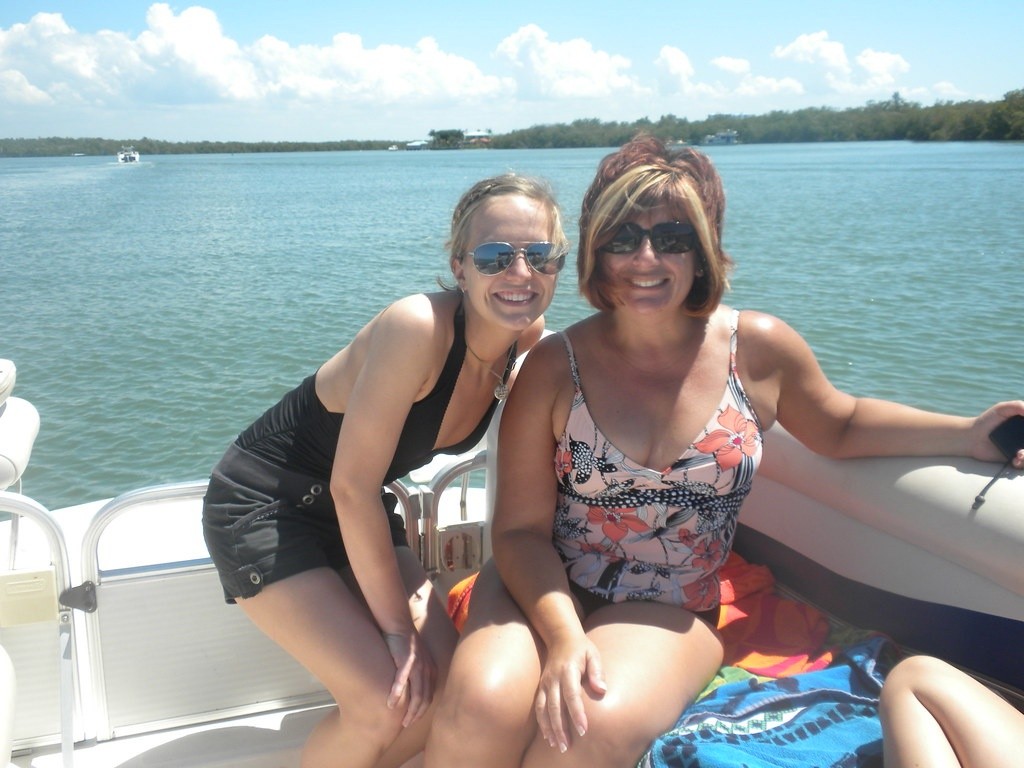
[601,221,695,254]
[463,240,565,276]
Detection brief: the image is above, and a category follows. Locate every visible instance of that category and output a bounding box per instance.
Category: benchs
[432,419,1024,768]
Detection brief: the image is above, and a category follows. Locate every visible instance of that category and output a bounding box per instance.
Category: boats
[0,419,1024,768]
[699,130,744,146]
[117,145,140,163]
[389,145,398,151]
[666,138,688,146]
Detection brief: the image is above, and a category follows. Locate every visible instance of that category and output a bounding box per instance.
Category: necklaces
[465,339,513,400]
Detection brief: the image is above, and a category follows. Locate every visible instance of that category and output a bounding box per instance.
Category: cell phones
[989,414,1024,460]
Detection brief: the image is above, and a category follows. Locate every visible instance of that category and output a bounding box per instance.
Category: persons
[202,171,570,768]
[878,654,1024,768]
[425,131,1024,768]
